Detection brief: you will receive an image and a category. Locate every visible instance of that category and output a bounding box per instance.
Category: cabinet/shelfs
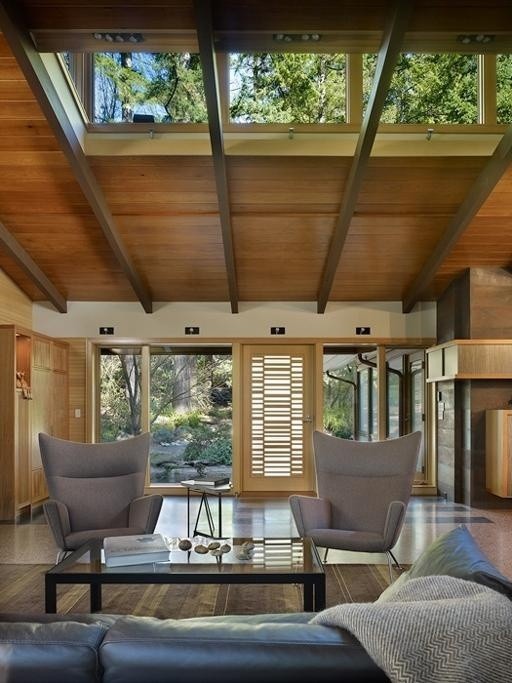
[0,321,70,525]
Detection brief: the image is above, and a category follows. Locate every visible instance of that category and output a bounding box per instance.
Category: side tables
[180,479,232,539]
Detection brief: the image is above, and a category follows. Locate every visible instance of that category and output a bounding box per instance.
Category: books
[102,533,170,567]
[194,476,229,486]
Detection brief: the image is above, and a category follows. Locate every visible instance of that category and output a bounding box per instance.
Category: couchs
[0,524,512,683]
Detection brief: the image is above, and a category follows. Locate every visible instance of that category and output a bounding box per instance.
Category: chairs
[33,429,165,566]
[285,426,419,587]
